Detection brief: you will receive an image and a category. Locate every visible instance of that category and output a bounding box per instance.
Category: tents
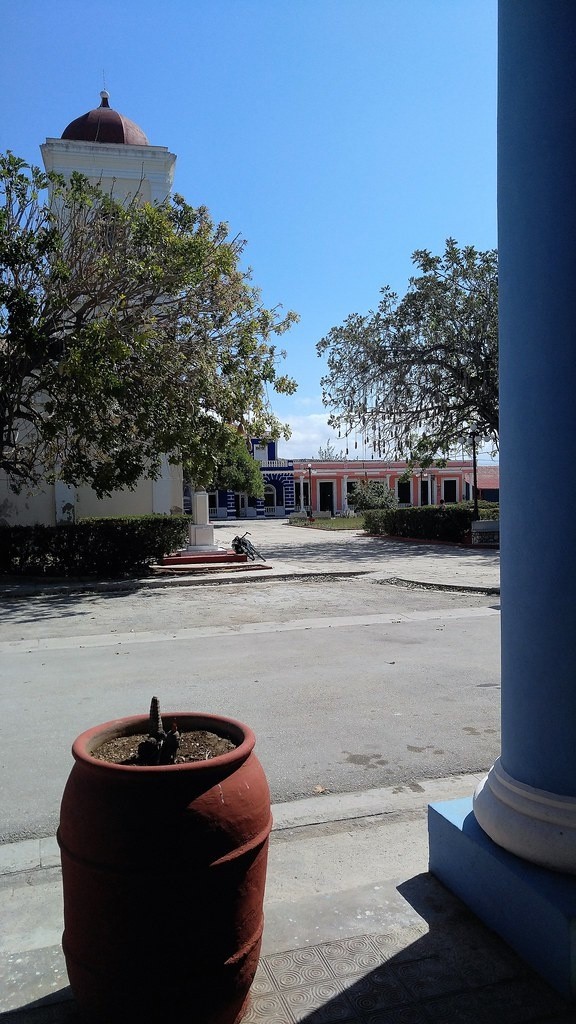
[466,474,499,500]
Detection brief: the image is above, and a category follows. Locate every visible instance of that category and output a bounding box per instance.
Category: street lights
[308,463,315,518]
[457,423,492,521]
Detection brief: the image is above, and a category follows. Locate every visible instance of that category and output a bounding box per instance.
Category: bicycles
[235,532,267,562]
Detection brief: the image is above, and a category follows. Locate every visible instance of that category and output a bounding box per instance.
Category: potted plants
[56,697,272,1024]
